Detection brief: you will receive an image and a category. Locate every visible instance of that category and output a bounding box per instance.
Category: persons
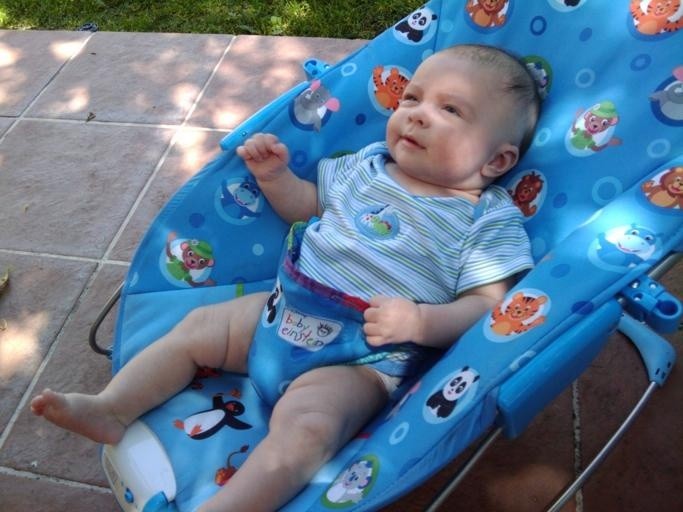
[29,44,541,512]
